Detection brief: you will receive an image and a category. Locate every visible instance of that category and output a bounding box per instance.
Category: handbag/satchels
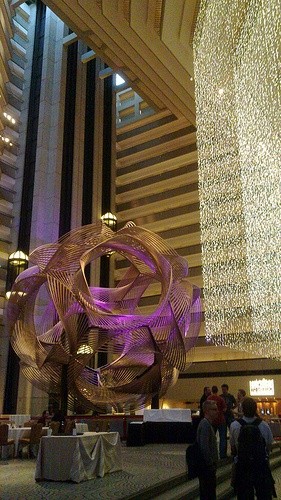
[185,444,213,477]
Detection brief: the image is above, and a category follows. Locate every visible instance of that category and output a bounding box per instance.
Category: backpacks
[235,418,267,464]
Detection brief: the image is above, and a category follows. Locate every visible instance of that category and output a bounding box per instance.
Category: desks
[10,427,49,438]
[34,431,124,484]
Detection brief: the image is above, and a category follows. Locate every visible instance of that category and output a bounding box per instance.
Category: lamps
[101,213,117,256]
[5,250,29,300]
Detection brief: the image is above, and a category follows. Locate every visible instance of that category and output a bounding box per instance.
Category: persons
[37,410,49,428]
[230,397,273,500]
[219,384,237,439]
[208,385,229,459]
[231,389,246,420]
[200,387,211,419]
[193,399,221,500]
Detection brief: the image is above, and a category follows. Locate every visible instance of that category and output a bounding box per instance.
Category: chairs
[0,423,16,460]
[17,424,43,458]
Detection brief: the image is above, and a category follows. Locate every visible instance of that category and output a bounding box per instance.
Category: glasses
[209,408,218,411]
